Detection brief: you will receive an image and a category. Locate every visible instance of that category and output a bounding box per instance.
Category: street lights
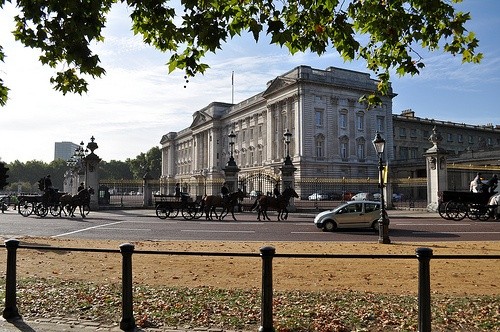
[372,130,391,244]
[65,141,88,191]
[226,129,239,169]
[281,128,295,168]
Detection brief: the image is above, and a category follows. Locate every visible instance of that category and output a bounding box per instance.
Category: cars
[308,191,401,202]
[314,200,389,232]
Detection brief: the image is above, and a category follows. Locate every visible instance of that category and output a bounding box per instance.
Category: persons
[355,204,360,212]
[220,182,228,199]
[78,183,84,192]
[175,183,180,195]
[274,184,280,197]
[45,175,52,189]
[474,172,498,192]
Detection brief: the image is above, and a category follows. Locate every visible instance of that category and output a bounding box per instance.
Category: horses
[59,186,95,218]
[250,185,299,221]
[200,189,245,220]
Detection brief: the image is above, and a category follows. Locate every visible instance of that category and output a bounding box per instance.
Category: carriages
[16,185,94,218]
[437,189,500,222]
[153,188,244,221]
